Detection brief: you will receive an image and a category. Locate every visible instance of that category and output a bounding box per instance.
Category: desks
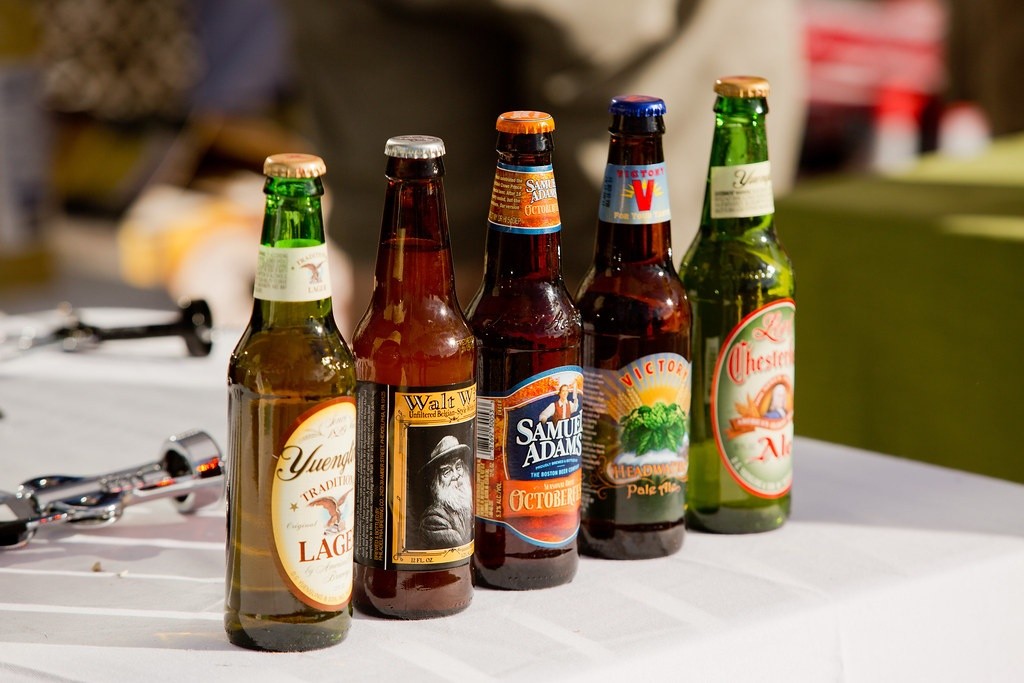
[0,304,1024,683]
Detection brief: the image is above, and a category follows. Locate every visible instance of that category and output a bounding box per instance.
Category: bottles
[572,93,692,559]
[466,110,579,593]
[678,76,792,532]
[353,136,479,620]
[224,155,354,654]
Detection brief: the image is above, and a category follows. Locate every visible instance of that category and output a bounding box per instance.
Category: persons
[539,384,579,427]
[418,435,473,550]
[116,0,811,358]
